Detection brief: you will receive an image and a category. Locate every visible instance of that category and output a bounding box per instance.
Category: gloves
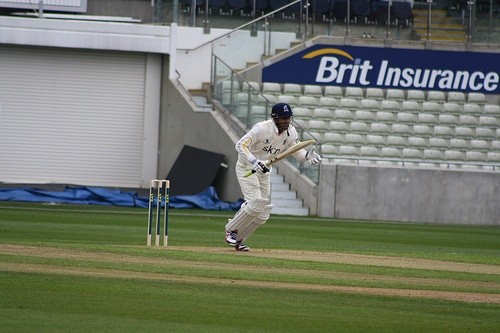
[305,146,322,165]
[252,159,270,175]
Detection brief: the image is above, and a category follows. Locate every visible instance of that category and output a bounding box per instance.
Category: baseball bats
[243,141,311,177]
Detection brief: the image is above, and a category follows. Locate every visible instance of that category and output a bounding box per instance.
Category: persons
[224,103,321,251]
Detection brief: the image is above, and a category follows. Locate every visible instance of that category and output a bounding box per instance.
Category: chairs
[223,80,500,171]
[183,0,414,27]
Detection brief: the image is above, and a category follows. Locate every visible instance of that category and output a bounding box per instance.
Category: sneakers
[236,242,249,251]
[224,231,238,247]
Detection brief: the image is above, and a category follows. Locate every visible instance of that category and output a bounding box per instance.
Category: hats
[270,103,294,117]
